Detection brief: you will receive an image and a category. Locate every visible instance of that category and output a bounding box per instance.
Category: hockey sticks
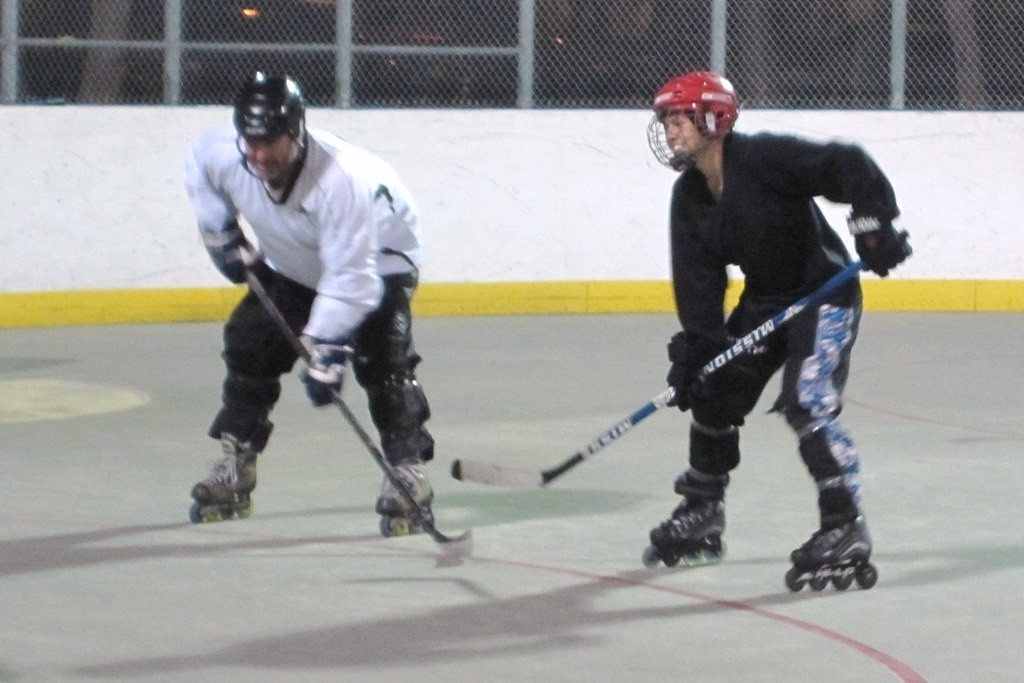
[242,265,474,566]
[449,230,912,490]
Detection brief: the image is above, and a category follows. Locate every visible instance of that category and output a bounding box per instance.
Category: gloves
[202,223,254,284]
[306,338,345,405]
[666,336,733,409]
[854,219,913,276]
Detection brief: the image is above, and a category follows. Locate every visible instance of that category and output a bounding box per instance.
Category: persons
[644,70,912,591]
[182,71,434,539]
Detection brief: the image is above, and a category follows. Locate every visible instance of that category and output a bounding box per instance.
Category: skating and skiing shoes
[787,516,877,594]
[644,495,725,568]
[376,454,434,537]
[189,432,258,523]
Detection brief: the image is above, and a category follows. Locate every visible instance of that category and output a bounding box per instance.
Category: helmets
[646,71,739,172]
[233,72,306,187]
